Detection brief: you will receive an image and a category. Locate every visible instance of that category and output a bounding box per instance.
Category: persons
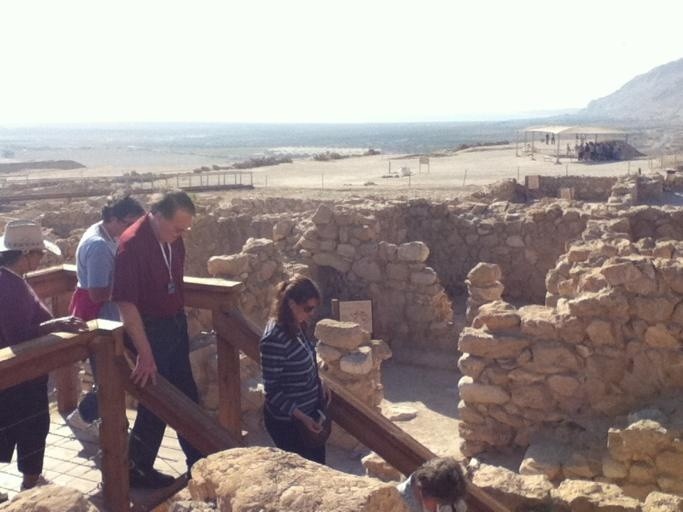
[546,133,556,144]
[575,140,621,162]
[509,491,575,511]
[109,188,196,488]
[257,274,333,467]
[392,454,468,511]
[66,195,147,431]
[0,220,90,503]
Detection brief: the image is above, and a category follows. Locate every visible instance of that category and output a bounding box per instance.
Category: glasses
[304,306,313,313]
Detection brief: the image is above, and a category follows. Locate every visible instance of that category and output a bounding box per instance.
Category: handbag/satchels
[290,410,330,446]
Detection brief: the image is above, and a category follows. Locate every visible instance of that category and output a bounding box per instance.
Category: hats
[0,220,61,256]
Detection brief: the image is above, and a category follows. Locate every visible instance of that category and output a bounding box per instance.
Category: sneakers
[129,461,174,488]
[20,475,53,491]
[67,408,93,430]
